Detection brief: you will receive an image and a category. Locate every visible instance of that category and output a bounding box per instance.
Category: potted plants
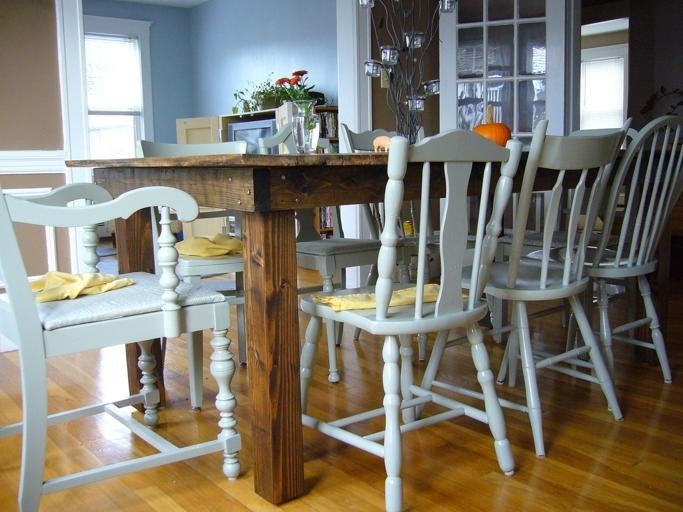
[231,79,291,114]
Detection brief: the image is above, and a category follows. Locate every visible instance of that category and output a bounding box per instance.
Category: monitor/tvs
[228,119,279,155]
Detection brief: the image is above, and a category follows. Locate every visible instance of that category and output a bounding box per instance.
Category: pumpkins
[472,102,511,147]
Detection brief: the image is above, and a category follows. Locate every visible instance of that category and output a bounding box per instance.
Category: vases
[292,100,321,155]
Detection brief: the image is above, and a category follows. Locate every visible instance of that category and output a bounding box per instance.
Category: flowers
[276,71,317,129]
